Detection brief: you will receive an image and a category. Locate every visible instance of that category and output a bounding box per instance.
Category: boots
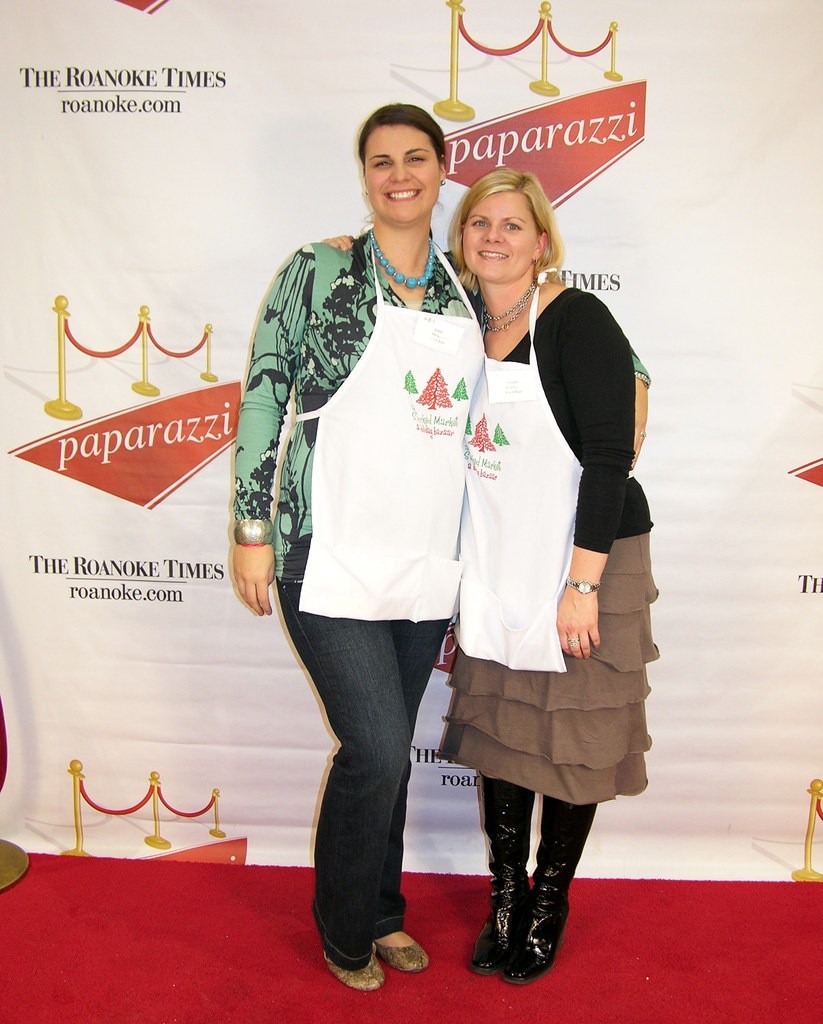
[470,777,535,975]
[503,795,597,983]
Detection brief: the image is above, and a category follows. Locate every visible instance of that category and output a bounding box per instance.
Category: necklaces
[369,227,436,288]
[483,280,538,332]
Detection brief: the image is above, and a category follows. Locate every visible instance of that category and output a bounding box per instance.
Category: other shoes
[324,949,385,991]
[374,931,429,973]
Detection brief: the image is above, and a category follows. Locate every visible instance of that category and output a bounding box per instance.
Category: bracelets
[634,371,650,389]
[234,519,274,545]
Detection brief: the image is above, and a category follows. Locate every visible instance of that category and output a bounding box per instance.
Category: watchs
[566,576,602,594]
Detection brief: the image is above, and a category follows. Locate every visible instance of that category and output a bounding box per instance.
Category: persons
[322,170,660,983]
[234,104,649,988]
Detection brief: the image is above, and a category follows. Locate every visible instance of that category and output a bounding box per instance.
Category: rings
[640,432,646,437]
[567,637,580,646]
